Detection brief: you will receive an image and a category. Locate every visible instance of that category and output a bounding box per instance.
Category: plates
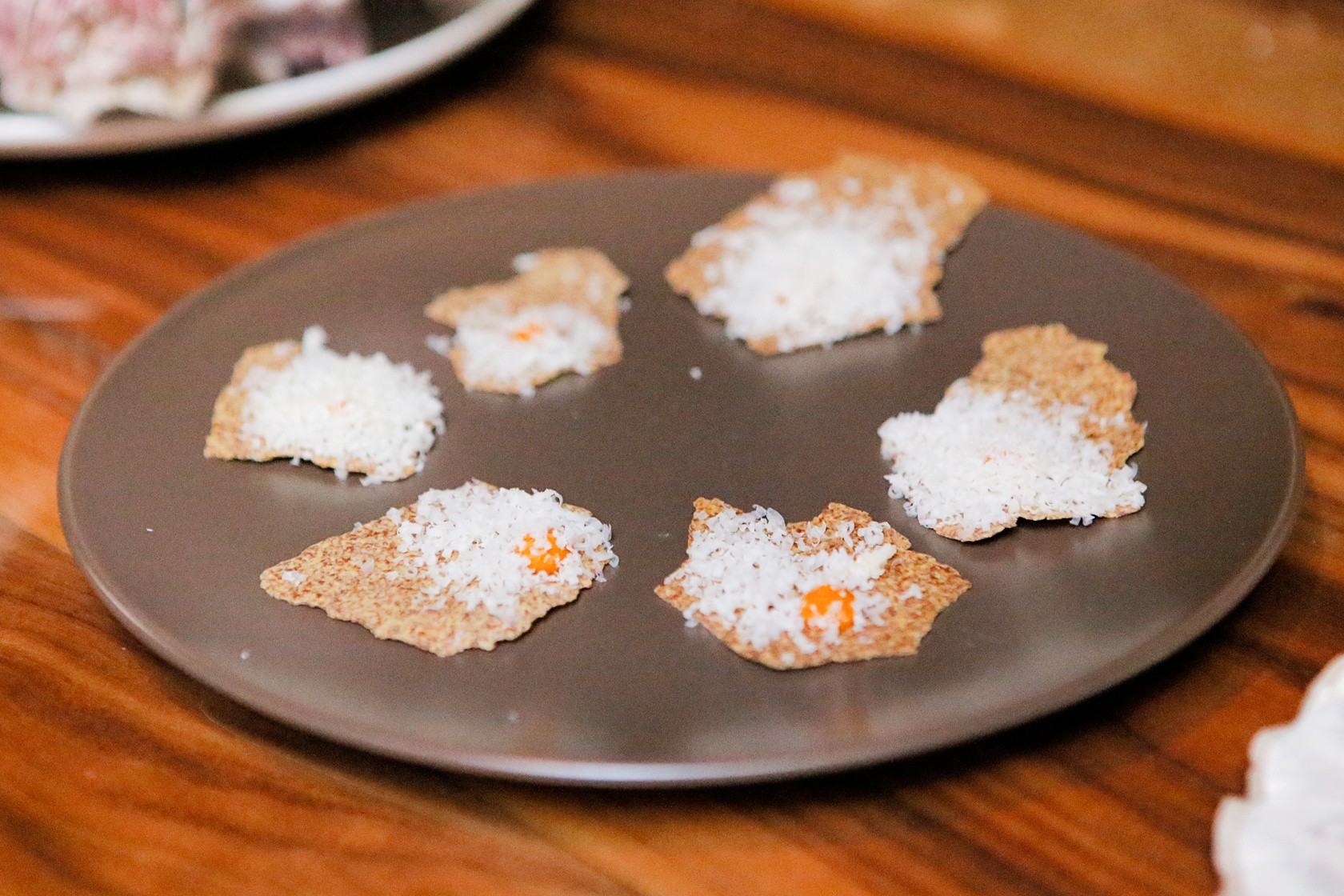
[2,0,534,162]
[54,169,1304,792]
